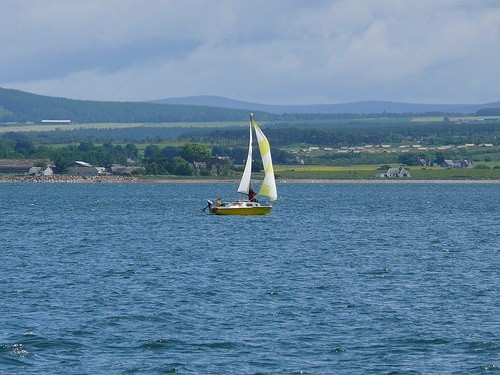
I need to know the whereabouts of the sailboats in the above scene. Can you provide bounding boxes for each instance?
[206,113,277,216]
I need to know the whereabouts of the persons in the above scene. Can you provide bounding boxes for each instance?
[248,187,259,203]
